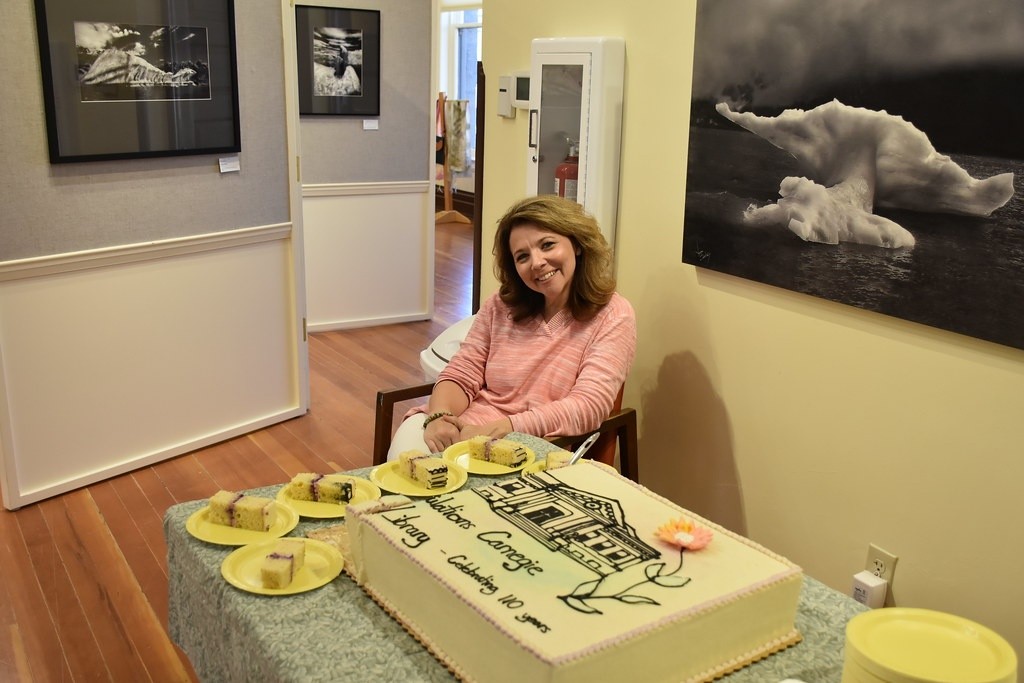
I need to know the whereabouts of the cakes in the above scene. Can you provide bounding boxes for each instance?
[545,450,575,469]
[468,434,527,468]
[208,489,278,533]
[258,539,305,590]
[289,472,358,505]
[397,449,448,489]
[346,459,805,683]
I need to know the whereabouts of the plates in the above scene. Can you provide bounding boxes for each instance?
[276,475,381,519]
[185,503,299,546]
[442,440,535,475]
[371,460,468,496]
[842,607,1019,683]
[521,459,617,477]
[221,537,344,595]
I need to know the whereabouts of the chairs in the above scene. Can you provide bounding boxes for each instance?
[372,376,639,484]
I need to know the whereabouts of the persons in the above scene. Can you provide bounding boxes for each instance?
[387,194,636,460]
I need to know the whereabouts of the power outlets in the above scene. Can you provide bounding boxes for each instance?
[867,543,898,594]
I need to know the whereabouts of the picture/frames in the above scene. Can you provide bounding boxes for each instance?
[34,0,242,165]
[294,3,381,117]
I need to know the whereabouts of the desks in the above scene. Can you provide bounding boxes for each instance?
[162,431,876,683]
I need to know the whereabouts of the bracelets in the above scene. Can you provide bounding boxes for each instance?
[423,411,453,428]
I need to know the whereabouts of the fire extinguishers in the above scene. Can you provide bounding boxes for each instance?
[554,136,579,201]
